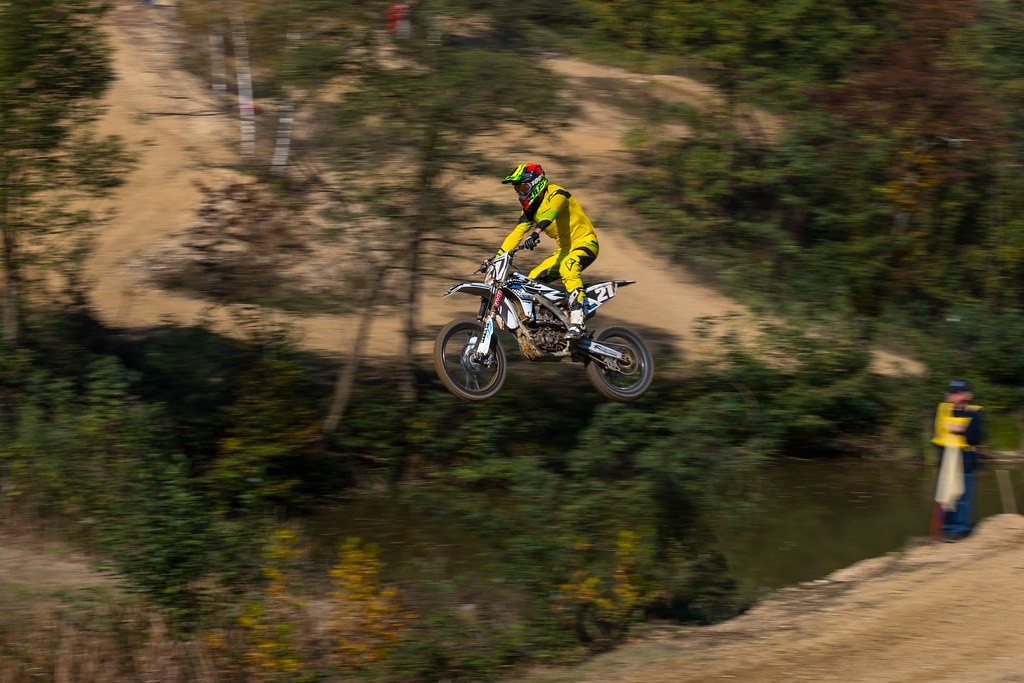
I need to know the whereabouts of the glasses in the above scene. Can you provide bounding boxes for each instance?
[514,171,545,196]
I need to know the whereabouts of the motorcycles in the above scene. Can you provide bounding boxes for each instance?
[433,236,656,404]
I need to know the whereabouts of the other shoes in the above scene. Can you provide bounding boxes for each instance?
[943,531,970,542]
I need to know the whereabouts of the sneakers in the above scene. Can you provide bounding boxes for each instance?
[508,320,539,333]
[564,324,589,340]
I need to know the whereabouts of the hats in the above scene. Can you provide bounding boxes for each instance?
[947,378,973,393]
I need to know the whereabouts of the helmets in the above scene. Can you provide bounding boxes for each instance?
[502,163,548,210]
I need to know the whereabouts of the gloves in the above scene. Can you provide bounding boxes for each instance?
[524,232,540,251]
[480,258,492,273]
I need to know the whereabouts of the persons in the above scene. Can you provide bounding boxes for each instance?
[930,380,984,543]
[479,163,599,340]
